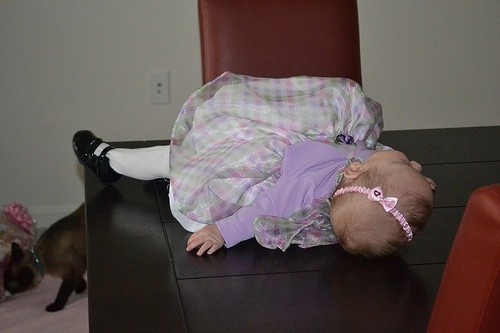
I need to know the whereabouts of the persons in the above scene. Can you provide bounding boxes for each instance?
[72,72,438,257]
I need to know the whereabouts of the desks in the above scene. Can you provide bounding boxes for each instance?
[84,126,500,333]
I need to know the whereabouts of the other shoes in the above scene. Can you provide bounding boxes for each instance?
[72,129,123,184]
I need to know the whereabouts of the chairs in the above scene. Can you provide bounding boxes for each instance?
[198,0,362,88]
[426,182,500,333]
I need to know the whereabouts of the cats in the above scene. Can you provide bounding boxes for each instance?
[3,203,87,312]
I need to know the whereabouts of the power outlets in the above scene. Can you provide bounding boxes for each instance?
[147,71,171,104]
